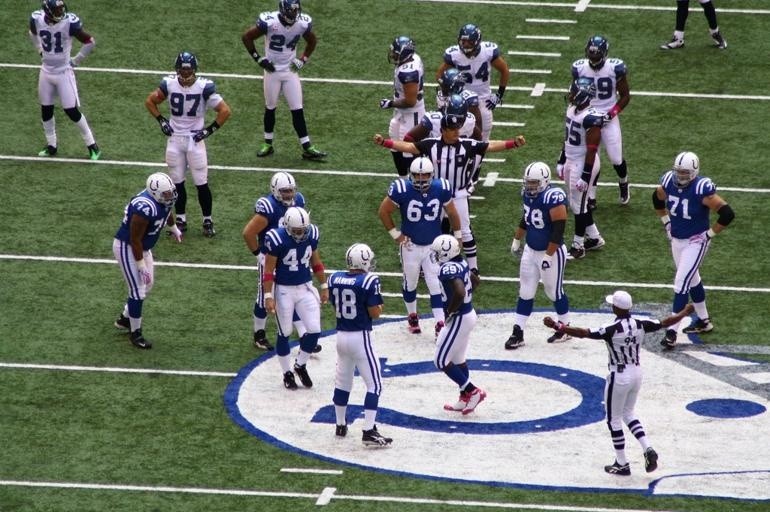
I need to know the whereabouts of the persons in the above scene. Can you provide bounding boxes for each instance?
[28,0,101,159]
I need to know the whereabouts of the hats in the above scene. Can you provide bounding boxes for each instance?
[605,289,633,311]
[439,114,465,130]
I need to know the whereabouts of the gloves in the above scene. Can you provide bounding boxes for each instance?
[509,243,525,262]
[539,256,553,272]
[379,98,391,110]
[688,230,708,247]
[665,221,675,243]
[190,128,213,143]
[555,163,565,182]
[575,179,590,195]
[169,224,183,244]
[484,93,502,112]
[292,58,306,73]
[258,55,278,74]
[138,266,154,286]
[159,114,175,139]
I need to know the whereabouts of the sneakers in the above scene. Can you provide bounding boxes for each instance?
[644,448,658,472]
[713,29,728,50]
[660,36,685,50]
[35,145,61,158]
[658,317,712,348]
[86,142,102,163]
[407,264,483,343]
[334,418,348,437]
[604,463,633,478]
[300,145,328,161]
[255,140,278,158]
[128,325,152,350]
[360,424,393,447]
[548,327,572,344]
[444,388,488,415]
[503,325,526,350]
[166,217,216,239]
[253,327,325,392]
[564,182,632,261]
[115,315,133,329]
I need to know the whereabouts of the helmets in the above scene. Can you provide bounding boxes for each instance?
[586,35,609,57]
[572,78,595,100]
[672,149,700,183]
[269,171,296,202]
[346,243,377,275]
[524,162,551,192]
[280,206,310,234]
[409,155,435,175]
[433,234,460,262]
[279,0,303,21]
[444,68,465,89]
[458,23,482,54]
[389,36,415,64]
[42,0,67,22]
[174,51,198,72]
[146,172,176,207]
